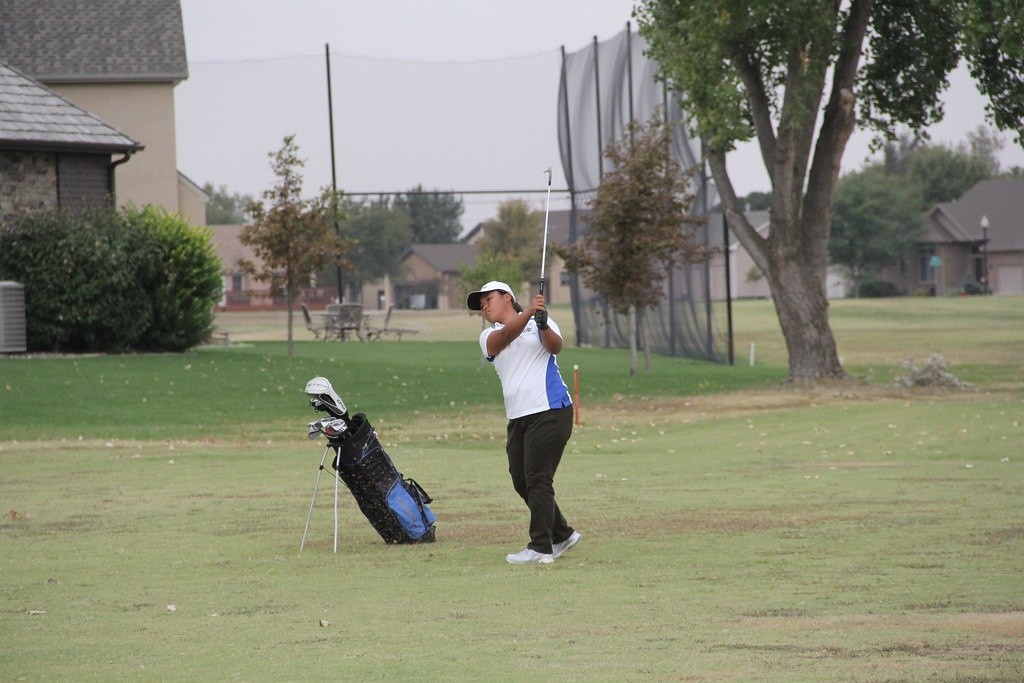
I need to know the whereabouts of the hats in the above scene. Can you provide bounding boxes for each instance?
[466,280,516,311]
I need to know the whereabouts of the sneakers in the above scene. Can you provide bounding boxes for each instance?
[551,532,580,558]
[506,546,553,563]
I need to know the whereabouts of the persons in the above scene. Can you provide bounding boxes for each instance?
[467,281,581,564]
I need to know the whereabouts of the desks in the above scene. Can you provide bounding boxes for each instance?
[315,312,374,341]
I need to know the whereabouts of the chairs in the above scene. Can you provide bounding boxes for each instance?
[300,302,419,343]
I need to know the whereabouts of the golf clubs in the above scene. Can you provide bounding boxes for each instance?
[536,166,554,327]
[306,416,352,440]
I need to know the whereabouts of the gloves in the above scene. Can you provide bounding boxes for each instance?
[534,307,549,330]
